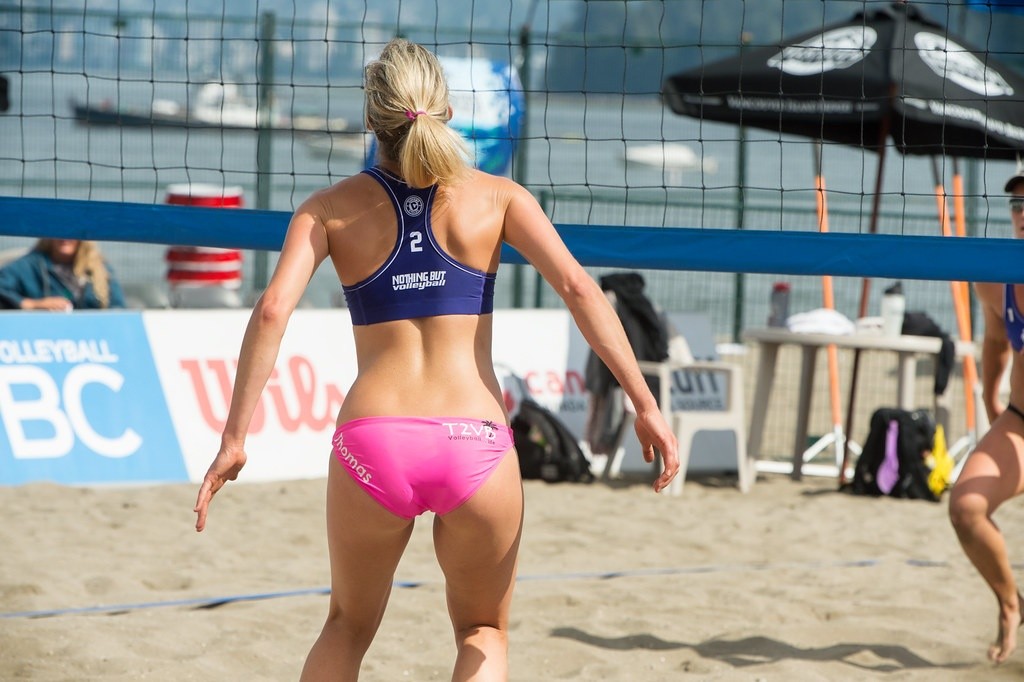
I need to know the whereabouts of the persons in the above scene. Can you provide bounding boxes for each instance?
[194,39,681,682]
[0,238,125,310]
[947,170,1024,662]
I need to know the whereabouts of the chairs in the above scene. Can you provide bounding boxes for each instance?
[600,291,747,498]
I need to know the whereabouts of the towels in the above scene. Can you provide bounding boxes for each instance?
[786,305,852,334]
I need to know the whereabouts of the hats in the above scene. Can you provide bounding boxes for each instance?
[1004,169,1024,192]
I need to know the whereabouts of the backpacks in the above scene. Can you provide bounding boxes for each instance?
[590,272,671,385]
[849,406,944,501]
[510,400,596,483]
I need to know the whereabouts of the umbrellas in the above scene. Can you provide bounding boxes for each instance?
[658,0,1024,487]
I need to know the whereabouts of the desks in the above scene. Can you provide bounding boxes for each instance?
[742,324,945,495]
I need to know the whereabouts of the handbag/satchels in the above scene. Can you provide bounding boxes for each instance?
[902,310,955,396]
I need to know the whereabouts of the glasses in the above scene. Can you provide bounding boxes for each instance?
[1009,198,1024,212]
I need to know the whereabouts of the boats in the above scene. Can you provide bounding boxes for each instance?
[616,140,717,176]
[67,89,348,136]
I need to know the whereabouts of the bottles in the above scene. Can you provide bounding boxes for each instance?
[767,283,791,330]
[880,279,906,335]
